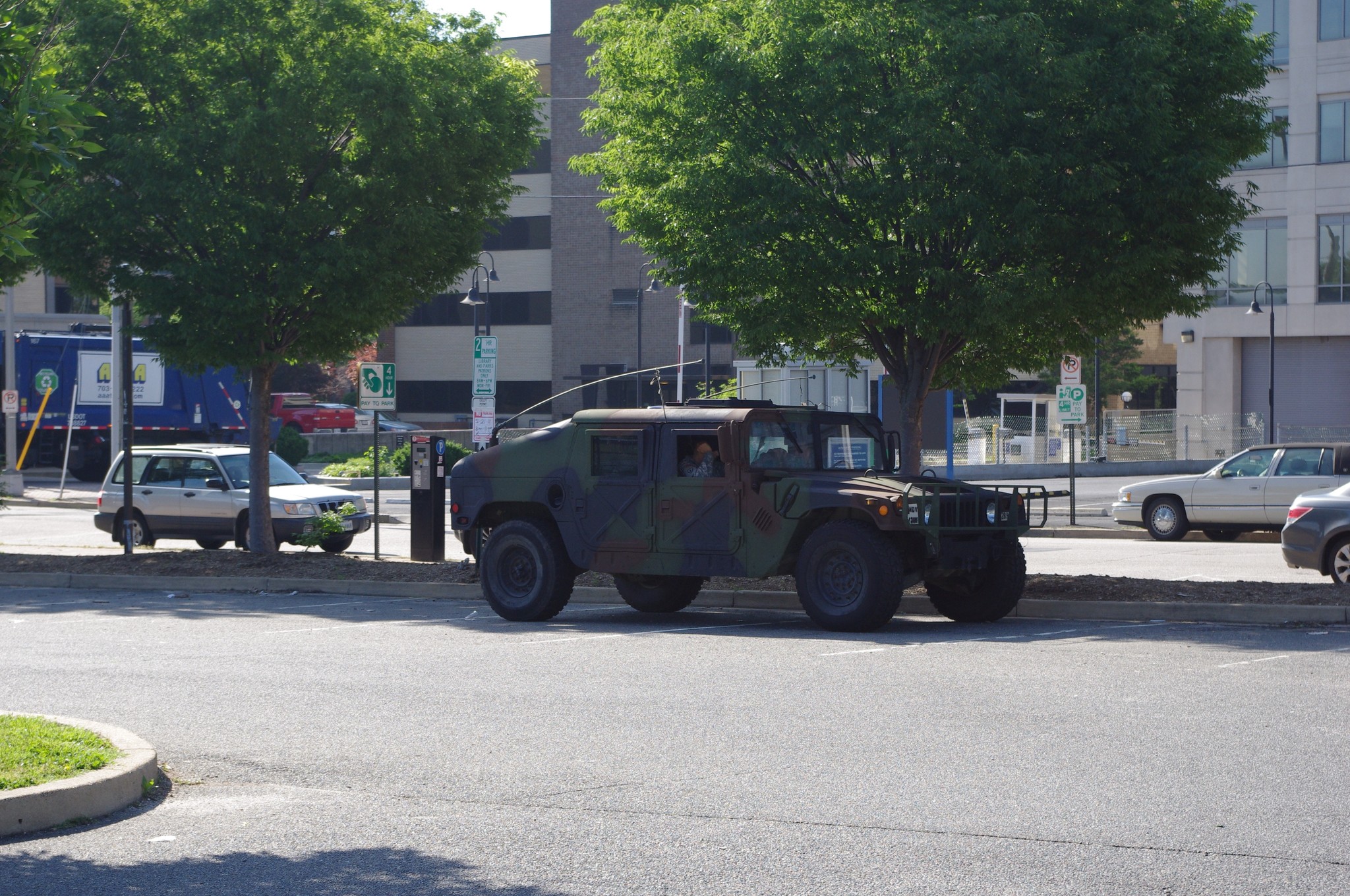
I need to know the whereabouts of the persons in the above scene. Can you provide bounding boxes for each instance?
[789,437,816,471]
[678,435,721,478]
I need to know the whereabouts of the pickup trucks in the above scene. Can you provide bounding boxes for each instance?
[267,392,354,433]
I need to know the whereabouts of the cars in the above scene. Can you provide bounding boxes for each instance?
[315,402,423,433]
[1111,441,1350,541]
[1281,481,1350,584]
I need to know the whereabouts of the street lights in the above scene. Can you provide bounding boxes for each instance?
[1244,281,1275,444]
[460,251,500,336]
[635,256,674,411]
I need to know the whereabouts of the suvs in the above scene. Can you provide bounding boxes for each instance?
[93,443,372,556]
[449,395,1050,630]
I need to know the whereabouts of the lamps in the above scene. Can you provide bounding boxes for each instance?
[1182,330,1196,345]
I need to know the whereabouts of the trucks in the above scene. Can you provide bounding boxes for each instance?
[14,322,280,483]
